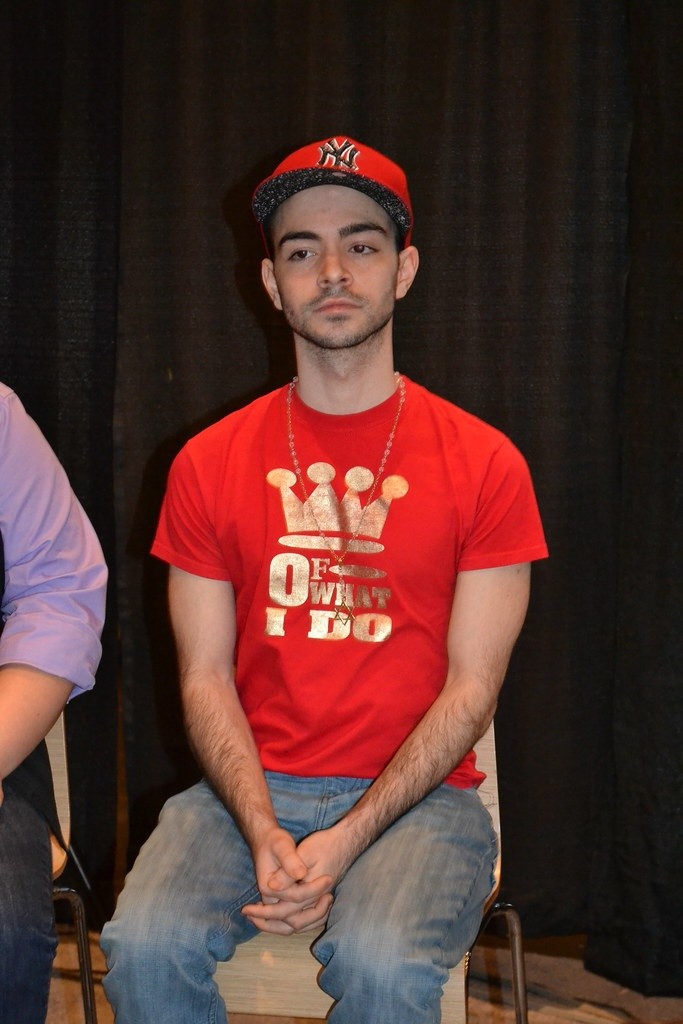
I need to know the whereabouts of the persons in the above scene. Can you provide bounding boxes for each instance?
[1,380,111,1024]
[101,134,549,1023]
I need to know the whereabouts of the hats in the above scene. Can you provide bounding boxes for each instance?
[251,137,414,260]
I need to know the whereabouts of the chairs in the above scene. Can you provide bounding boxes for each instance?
[211,715,529,1024]
[32,712,98,1024]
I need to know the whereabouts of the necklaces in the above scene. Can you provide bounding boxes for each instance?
[286,370,408,630]
